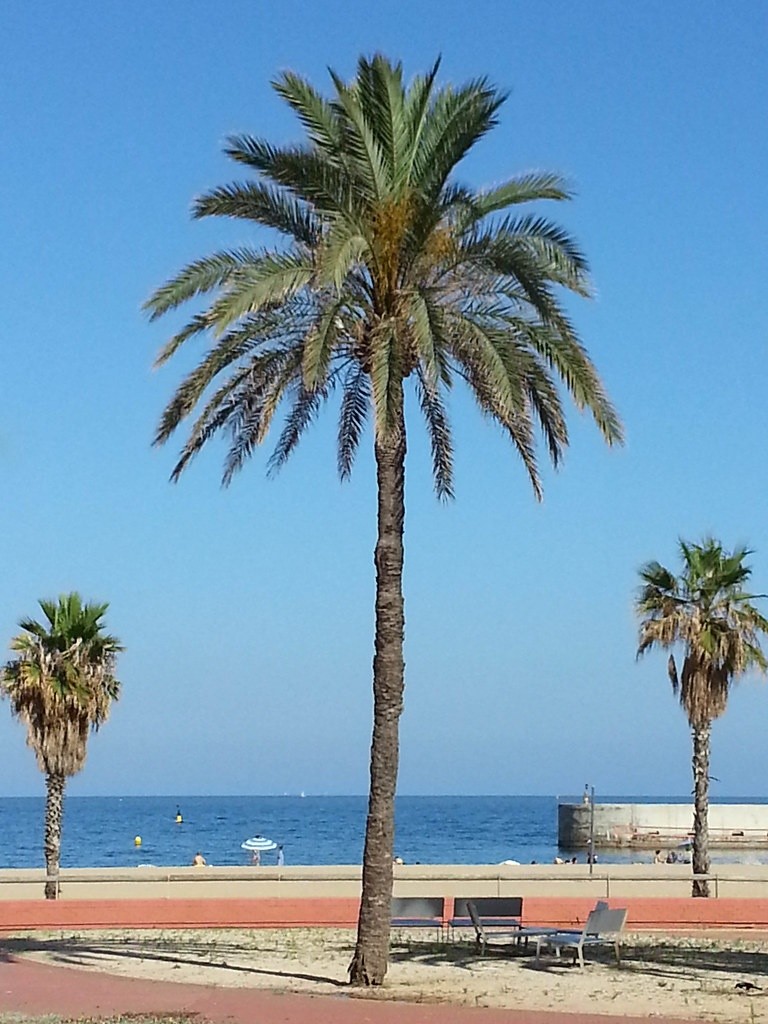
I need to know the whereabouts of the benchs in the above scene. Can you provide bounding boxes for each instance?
[447,897,523,947]
[390,897,446,949]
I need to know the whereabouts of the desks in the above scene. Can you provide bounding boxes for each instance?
[514,926,557,954]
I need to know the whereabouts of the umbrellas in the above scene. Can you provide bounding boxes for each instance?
[241,833,277,852]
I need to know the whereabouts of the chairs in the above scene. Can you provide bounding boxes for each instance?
[536,908,628,971]
[466,901,518,956]
[555,900,610,959]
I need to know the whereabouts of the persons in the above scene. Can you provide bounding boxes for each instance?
[193,852,207,867]
[252,846,286,866]
[393,850,693,865]
